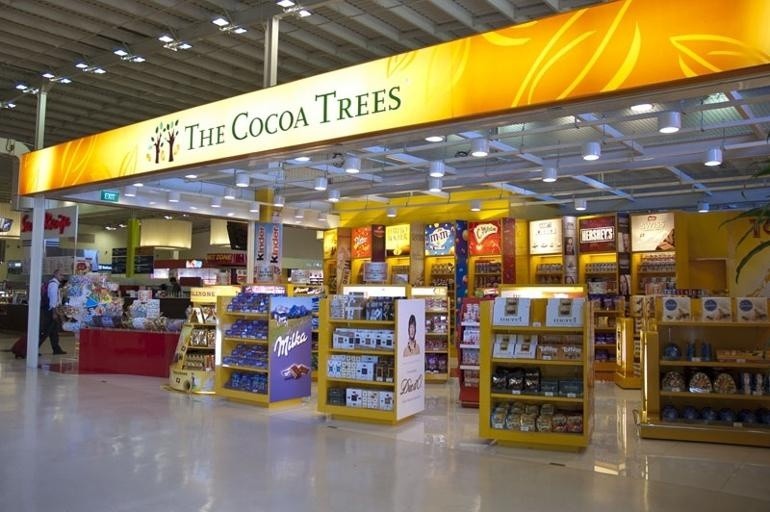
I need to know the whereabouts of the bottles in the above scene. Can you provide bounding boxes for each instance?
[538,262,616,272]
[688,341,712,361]
[0,292,9,304]
[639,254,675,271]
[432,263,454,274]
[184,354,204,370]
[475,259,490,273]
[393,265,409,273]
[664,286,678,295]
[343,292,367,320]
[168,290,183,298]
[540,275,560,284]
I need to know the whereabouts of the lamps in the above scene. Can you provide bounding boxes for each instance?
[124,109,725,218]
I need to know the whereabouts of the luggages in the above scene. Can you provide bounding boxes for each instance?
[11,330,43,359]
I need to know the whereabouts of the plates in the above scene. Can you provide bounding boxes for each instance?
[660,404,770,428]
[663,343,681,361]
[661,371,738,395]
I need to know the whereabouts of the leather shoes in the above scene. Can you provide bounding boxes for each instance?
[39,353,42,357]
[53,348,68,356]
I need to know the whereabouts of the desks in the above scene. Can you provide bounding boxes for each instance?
[0,293,191,378]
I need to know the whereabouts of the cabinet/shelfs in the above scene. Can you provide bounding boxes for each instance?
[459,219,594,456]
[169,208,465,428]
[578,212,770,448]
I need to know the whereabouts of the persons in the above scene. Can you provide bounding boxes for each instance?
[403,315,420,357]
[39,268,68,356]
[169,277,181,297]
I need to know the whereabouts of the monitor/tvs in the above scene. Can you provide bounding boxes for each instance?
[226,220,248,250]
